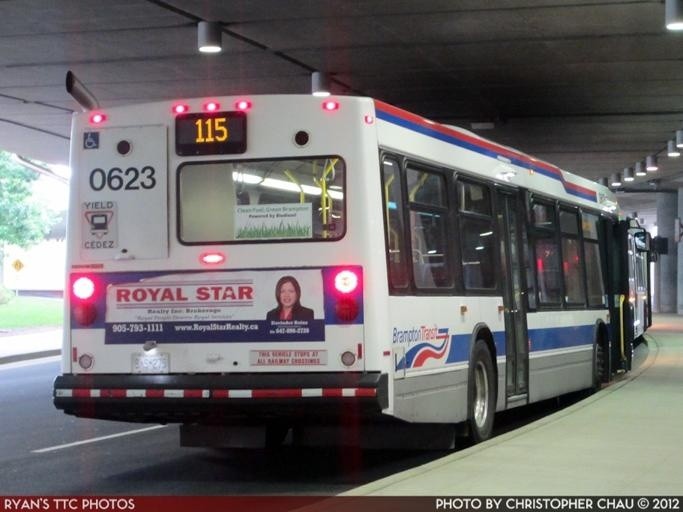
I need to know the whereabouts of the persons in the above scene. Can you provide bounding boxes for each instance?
[267,276,313,322]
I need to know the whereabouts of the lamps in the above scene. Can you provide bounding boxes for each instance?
[596,128,682,188]
[196,20,224,55]
[664,0,682,31]
[310,70,331,99]
